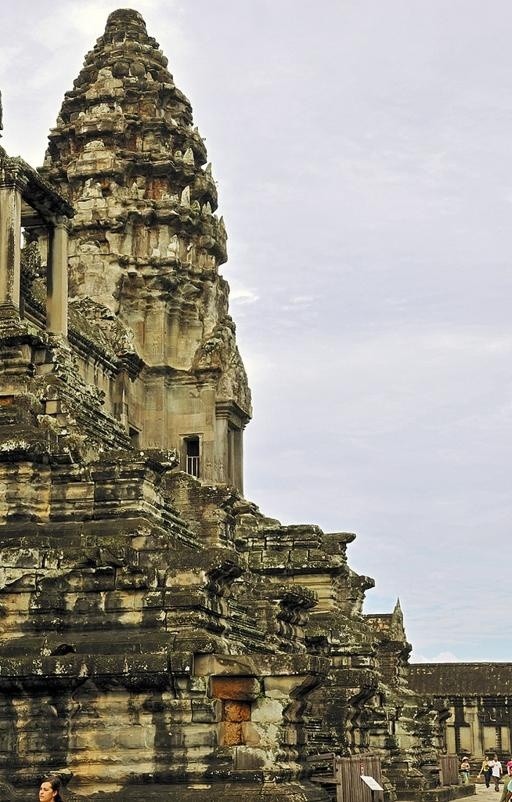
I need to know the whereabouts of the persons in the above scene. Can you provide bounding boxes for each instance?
[500,761,512,802]
[461,756,470,784]
[479,755,503,792]
[39,777,64,802]
